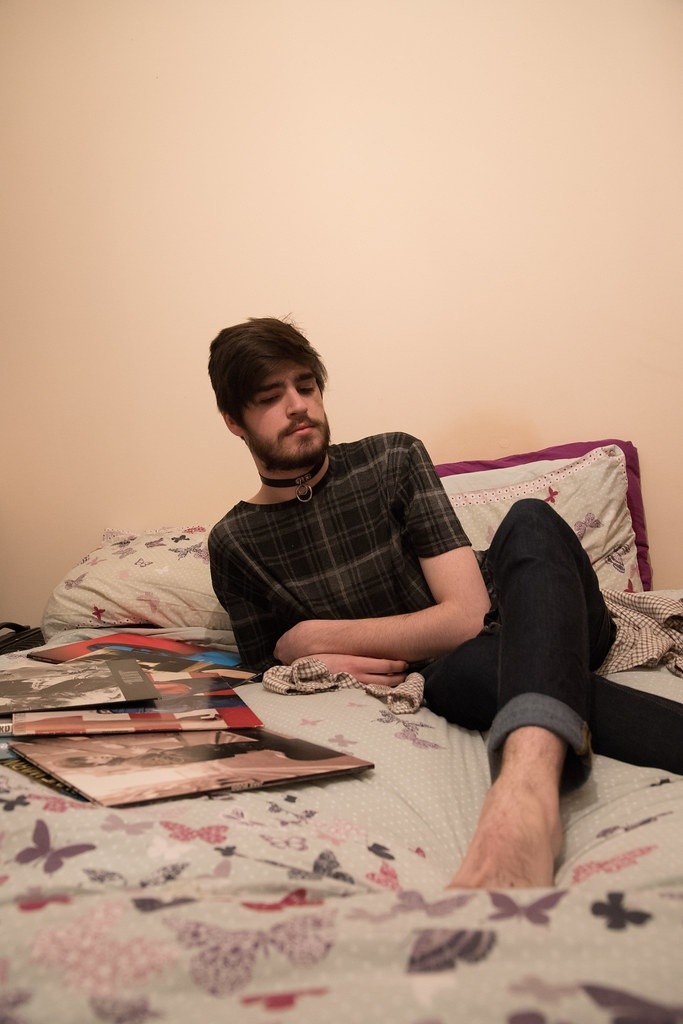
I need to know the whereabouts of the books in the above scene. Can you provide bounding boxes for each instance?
[0,632,376,810]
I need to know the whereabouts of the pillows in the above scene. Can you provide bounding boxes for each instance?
[40,526,235,642]
[435,440,654,594]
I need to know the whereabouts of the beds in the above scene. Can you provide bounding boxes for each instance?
[0,624,683,1024]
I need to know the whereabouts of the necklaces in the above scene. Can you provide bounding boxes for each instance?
[259,455,326,503]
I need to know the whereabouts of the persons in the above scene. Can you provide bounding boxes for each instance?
[207,316,683,889]
[97,691,248,714]
[58,735,281,768]
